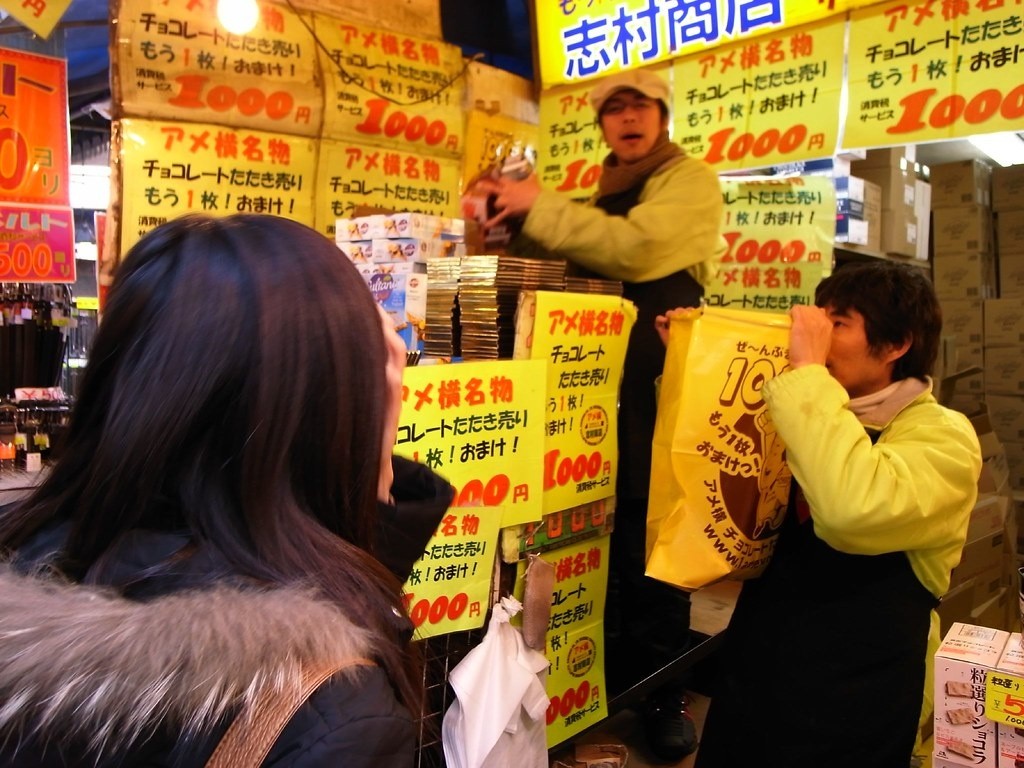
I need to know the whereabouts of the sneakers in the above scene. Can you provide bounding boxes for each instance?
[648,686,698,759]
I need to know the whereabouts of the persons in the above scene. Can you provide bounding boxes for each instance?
[694,263,982,768]
[0,214,455,768]
[484,70,721,758]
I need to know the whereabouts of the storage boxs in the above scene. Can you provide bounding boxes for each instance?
[333,204,467,366]
[779,143,1024,768]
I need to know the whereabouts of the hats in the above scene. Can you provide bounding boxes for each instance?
[589,67,671,111]
